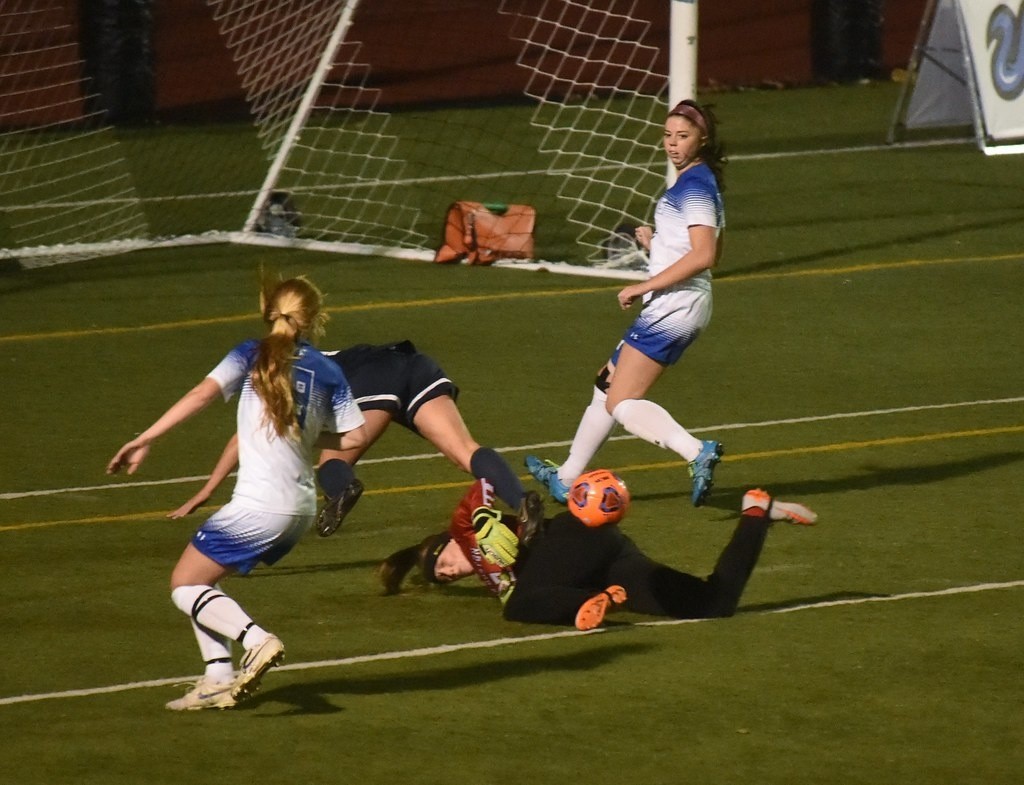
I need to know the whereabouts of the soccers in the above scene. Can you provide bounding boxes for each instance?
[569,469,630,529]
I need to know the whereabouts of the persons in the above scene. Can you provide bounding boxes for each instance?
[521,96,733,508]
[300,337,523,533]
[377,470,818,632]
[103,274,368,711]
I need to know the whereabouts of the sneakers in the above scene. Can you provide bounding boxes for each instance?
[233,635,286,701]
[739,487,818,525]
[522,454,569,504]
[687,439,725,507]
[574,584,628,629]
[163,679,239,710]
[313,478,364,535]
